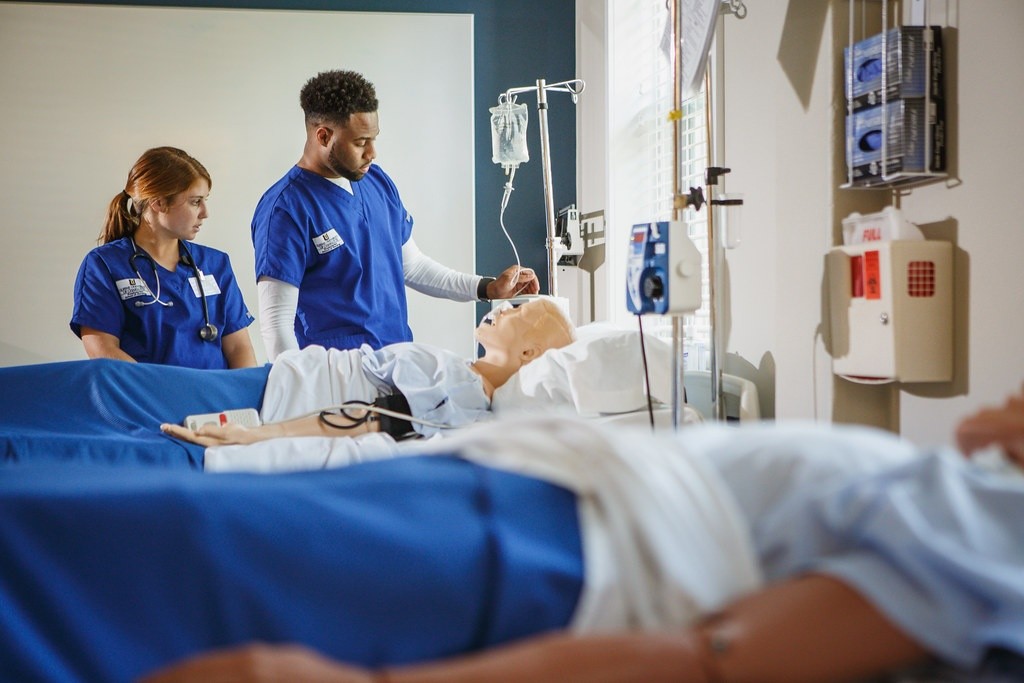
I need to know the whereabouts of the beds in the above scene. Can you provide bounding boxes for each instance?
[0,363,769,473]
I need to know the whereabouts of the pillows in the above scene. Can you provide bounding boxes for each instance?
[488,319,689,419]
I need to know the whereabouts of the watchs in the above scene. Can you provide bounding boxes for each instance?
[477,276,496,304]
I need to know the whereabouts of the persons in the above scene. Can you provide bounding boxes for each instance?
[0,296,578,449]
[1,381,1024,683]
[251,70,542,362]
[69,146,258,370]
